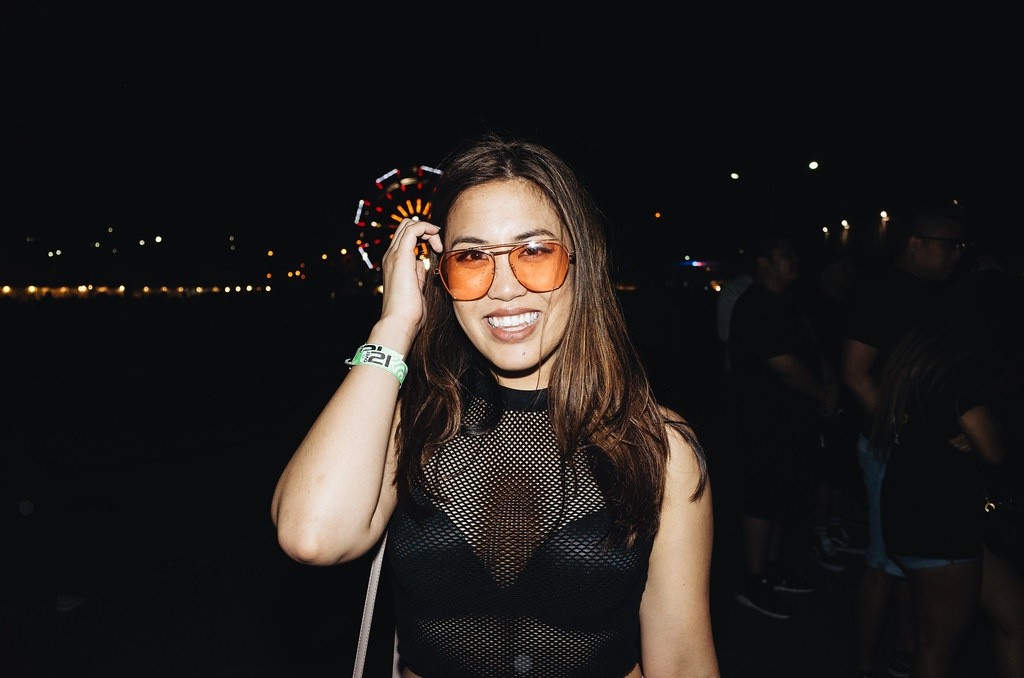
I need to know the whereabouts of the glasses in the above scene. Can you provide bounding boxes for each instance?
[433,239,577,301]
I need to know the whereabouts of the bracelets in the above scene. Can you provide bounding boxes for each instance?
[346,345,407,386]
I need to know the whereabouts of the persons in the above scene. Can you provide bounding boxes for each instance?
[715,208,1024,678]
[272,142,721,678]
